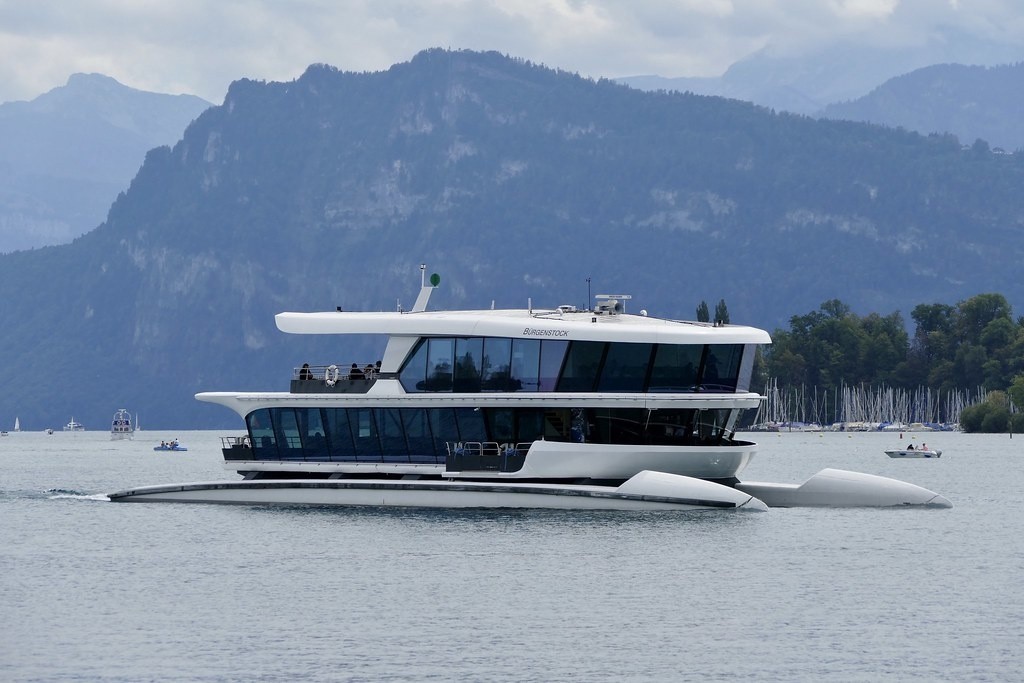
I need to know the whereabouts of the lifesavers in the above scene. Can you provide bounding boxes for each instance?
[325,364,339,386]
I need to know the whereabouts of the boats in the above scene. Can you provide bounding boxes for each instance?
[883,448,943,458]
[153,444,187,451]
[107,261,954,514]
[110,408,135,441]
[1,430,9,436]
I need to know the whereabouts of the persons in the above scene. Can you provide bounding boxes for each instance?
[375,360,382,373]
[300,364,313,380]
[349,363,366,380]
[161,438,179,449]
[922,443,928,451]
[907,443,914,451]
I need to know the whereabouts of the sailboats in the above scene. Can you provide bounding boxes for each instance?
[738,377,988,432]
[13,417,21,432]
[134,413,140,431]
